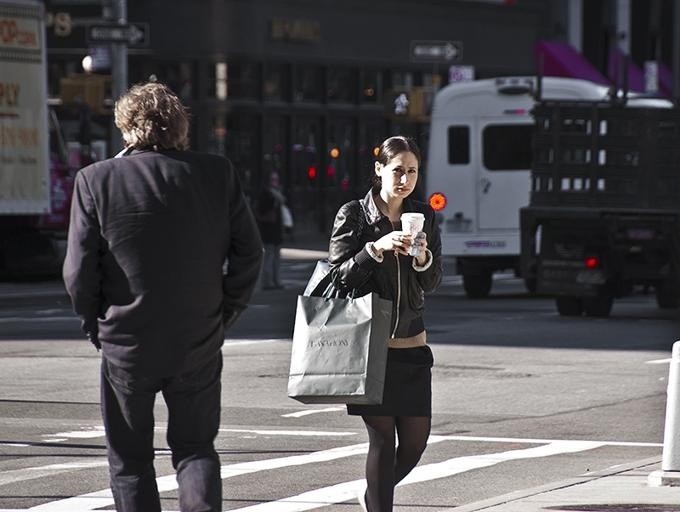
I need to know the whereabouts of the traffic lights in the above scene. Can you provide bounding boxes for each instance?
[387,86,424,123]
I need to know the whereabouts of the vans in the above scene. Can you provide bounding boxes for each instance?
[427,75,673,298]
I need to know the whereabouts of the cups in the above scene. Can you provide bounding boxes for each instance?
[400,212,426,254]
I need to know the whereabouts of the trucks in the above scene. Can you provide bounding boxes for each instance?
[519,56,680,317]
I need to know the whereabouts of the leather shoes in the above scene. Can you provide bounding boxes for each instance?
[358,487,368,512]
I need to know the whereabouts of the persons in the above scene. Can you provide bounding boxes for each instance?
[253,172,293,290]
[329,135,444,512]
[62,81,265,512]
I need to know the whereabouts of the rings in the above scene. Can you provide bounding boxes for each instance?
[398,236,401,241]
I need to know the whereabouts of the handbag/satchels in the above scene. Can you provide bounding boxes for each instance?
[287,261,393,407]
